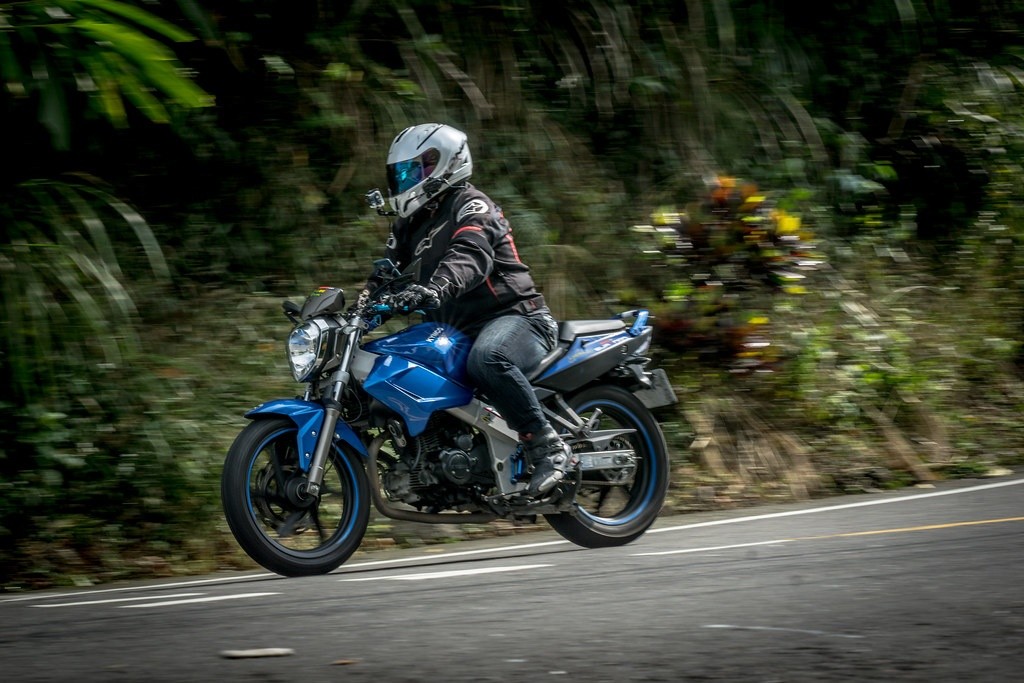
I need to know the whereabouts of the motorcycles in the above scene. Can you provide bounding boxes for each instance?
[222,260,682,577]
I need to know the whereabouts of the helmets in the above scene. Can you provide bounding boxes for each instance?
[385,122,473,219]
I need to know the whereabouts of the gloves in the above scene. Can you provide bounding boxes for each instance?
[386,284,438,314]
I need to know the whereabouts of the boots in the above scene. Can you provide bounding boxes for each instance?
[520,421,573,496]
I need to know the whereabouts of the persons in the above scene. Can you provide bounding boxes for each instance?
[361,123,571,495]
